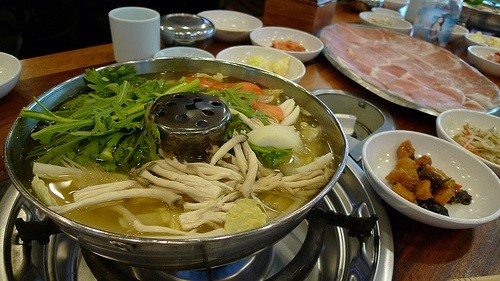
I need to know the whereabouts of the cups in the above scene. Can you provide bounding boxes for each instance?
[108,7,161,63]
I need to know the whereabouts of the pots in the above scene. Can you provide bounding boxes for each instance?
[3,57,349,271]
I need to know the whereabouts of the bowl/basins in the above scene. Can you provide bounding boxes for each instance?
[372,7,403,20]
[250,26,330,64]
[153,47,214,63]
[359,11,413,36]
[216,46,306,85]
[384,0,407,10]
[464,34,500,49]
[467,45,500,79]
[362,130,500,228]
[0,51,21,100]
[448,24,469,43]
[197,9,263,42]
[437,109,500,178]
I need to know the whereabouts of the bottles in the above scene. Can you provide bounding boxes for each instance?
[410,0,464,48]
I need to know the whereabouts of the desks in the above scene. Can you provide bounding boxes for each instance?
[0,0,500,281]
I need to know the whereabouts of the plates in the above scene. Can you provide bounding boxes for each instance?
[319,21,500,115]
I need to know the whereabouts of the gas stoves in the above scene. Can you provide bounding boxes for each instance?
[0,154,394,281]
